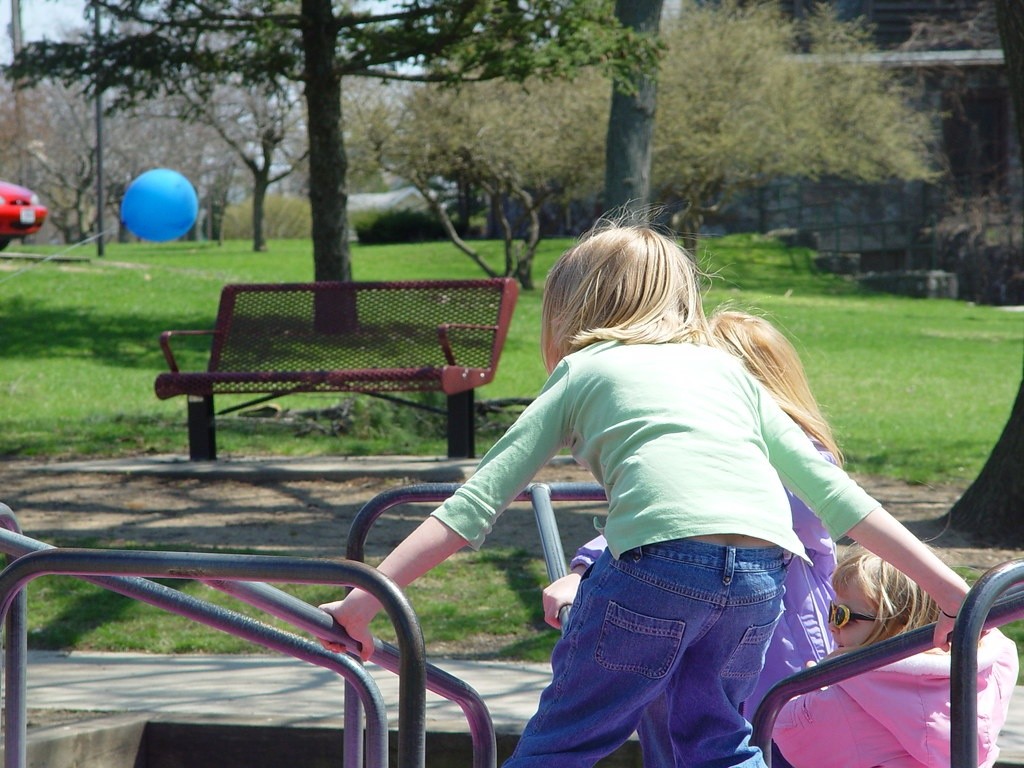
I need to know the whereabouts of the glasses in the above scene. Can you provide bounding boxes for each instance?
[827,601,876,628]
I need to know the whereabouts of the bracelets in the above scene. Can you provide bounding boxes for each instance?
[941,608,957,621]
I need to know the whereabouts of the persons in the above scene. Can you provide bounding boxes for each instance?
[315,197,969,768]
[541,298,846,768]
[771,517,1020,768]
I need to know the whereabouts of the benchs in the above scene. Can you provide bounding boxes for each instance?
[153,277,519,460]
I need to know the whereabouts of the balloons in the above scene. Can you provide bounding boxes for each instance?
[116,165,200,245]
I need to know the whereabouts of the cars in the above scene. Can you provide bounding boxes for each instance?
[0,180,49,253]
